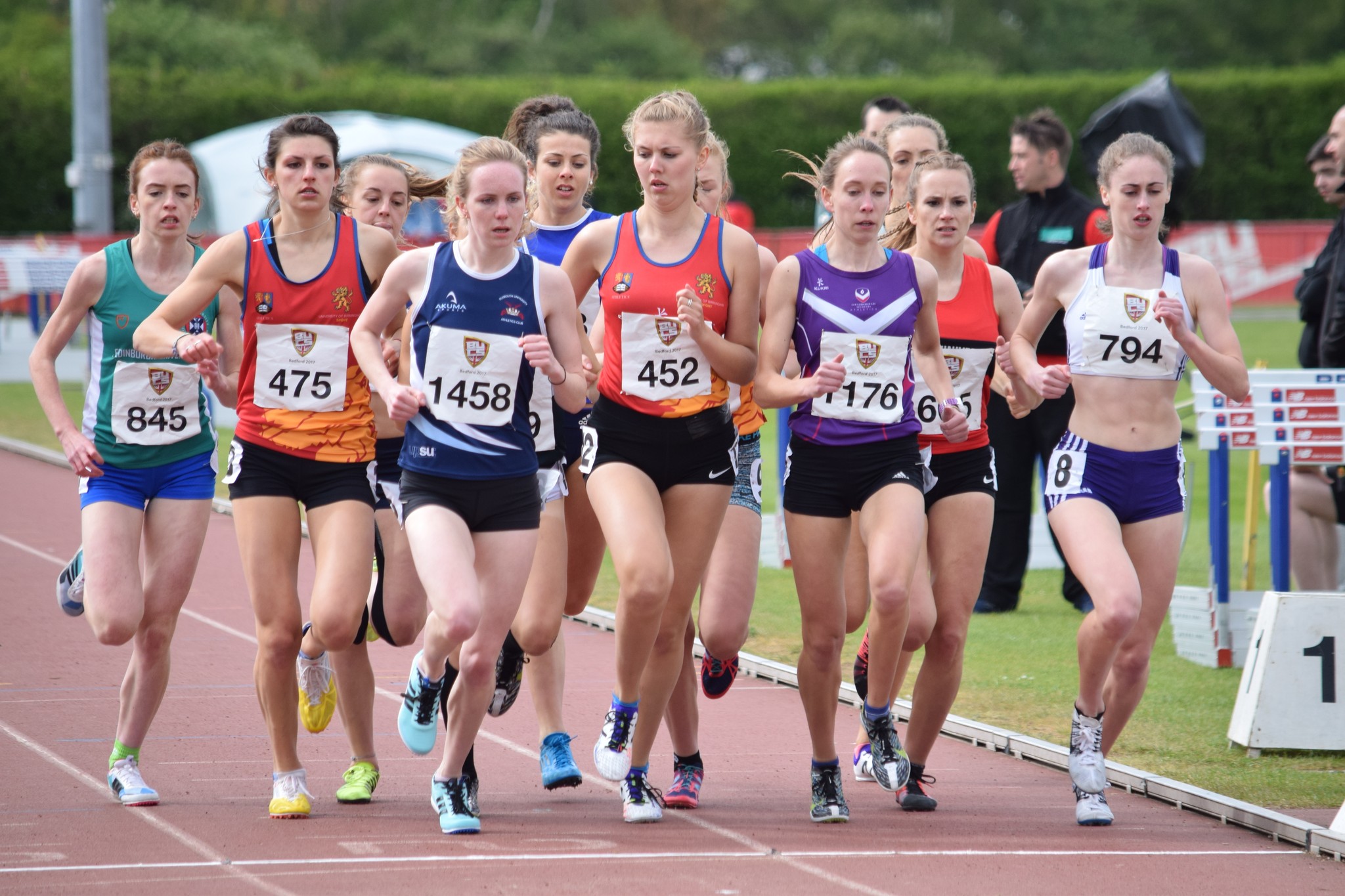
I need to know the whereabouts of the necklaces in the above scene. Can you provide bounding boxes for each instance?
[252,207,331,242]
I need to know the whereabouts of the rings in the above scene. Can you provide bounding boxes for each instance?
[184,344,195,350]
[387,359,391,368]
[686,298,692,307]
[194,340,204,346]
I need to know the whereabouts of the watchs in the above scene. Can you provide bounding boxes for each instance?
[938,397,969,417]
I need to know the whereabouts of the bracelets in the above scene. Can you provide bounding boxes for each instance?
[170,331,199,361]
[547,366,566,385]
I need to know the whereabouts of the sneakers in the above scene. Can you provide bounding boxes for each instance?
[488,647,530,718]
[619,769,668,823]
[297,620,338,733]
[335,762,380,804]
[895,770,938,811]
[859,693,911,791]
[56,543,84,617]
[810,765,849,822]
[397,648,446,755]
[852,744,878,781]
[1072,782,1115,827]
[268,768,315,819]
[662,757,704,809]
[429,767,482,835]
[592,700,639,780]
[700,647,739,699]
[853,626,870,699]
[1067,698,1106,794]
[107,754,159,807]
[538,731,582,792]
[365,555,381,643]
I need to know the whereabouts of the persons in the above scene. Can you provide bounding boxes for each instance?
[331,135,591,838]
[751,95,1115,827]
[694,138,728,216]
[1008,130,1250,827]
[1260,96,1345,608]
[26,140,246,809]
[496,89,783,830]
[132,114,400,820]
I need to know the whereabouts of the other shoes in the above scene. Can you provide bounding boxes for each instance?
[972,597,1019,613]
[1073,593,1095,616]
